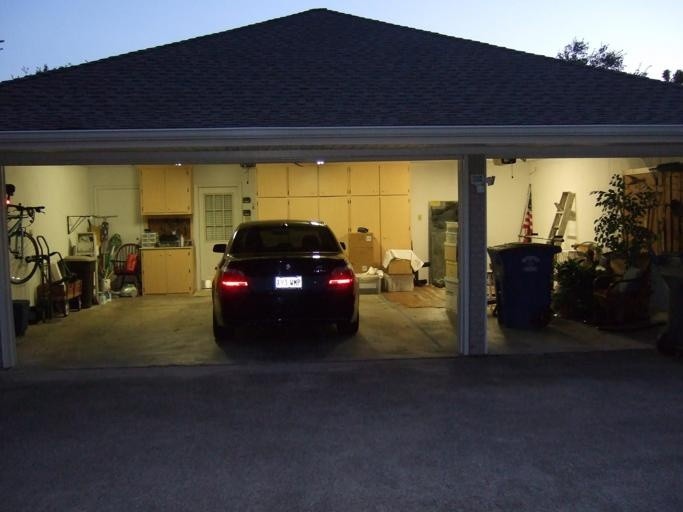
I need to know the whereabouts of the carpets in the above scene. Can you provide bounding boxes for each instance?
[383,285,446,308]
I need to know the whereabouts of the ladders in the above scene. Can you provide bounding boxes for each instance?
[546,192,576,262]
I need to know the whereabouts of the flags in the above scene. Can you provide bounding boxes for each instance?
[521,187,532,244]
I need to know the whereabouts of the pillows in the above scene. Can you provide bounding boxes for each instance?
[620,265,640,293]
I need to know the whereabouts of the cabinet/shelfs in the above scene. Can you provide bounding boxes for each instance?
[257,161,411,270]
[139,246,198,295]
[137,164,194,215]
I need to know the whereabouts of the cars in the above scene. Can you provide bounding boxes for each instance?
[208,217,361,343]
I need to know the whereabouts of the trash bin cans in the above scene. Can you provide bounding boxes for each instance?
[486,242,562,330]
[62,256,97,309]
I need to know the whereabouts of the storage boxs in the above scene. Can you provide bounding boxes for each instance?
[357,249,414,293]
[444,221,458,278]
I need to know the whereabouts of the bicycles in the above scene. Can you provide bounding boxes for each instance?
[6,201,45,285]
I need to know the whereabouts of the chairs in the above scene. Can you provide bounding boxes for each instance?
[591,253,653,323]
